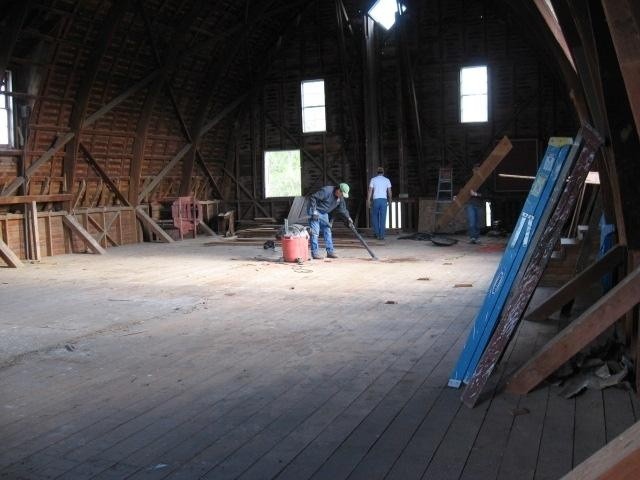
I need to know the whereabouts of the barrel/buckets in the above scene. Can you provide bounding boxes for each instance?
[281,234,309,262]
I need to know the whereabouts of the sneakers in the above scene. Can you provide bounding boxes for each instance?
[313,253,325,259]
[327,253,339,258]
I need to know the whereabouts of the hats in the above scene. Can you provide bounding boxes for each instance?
[336,182,350,199]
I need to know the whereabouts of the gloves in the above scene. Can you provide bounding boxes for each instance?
[312,212,320,222]
[344,218,353,228]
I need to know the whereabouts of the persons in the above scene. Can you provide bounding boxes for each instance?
[306,181,354,261]
[453,162,497,245]
[364,166,395,242]
